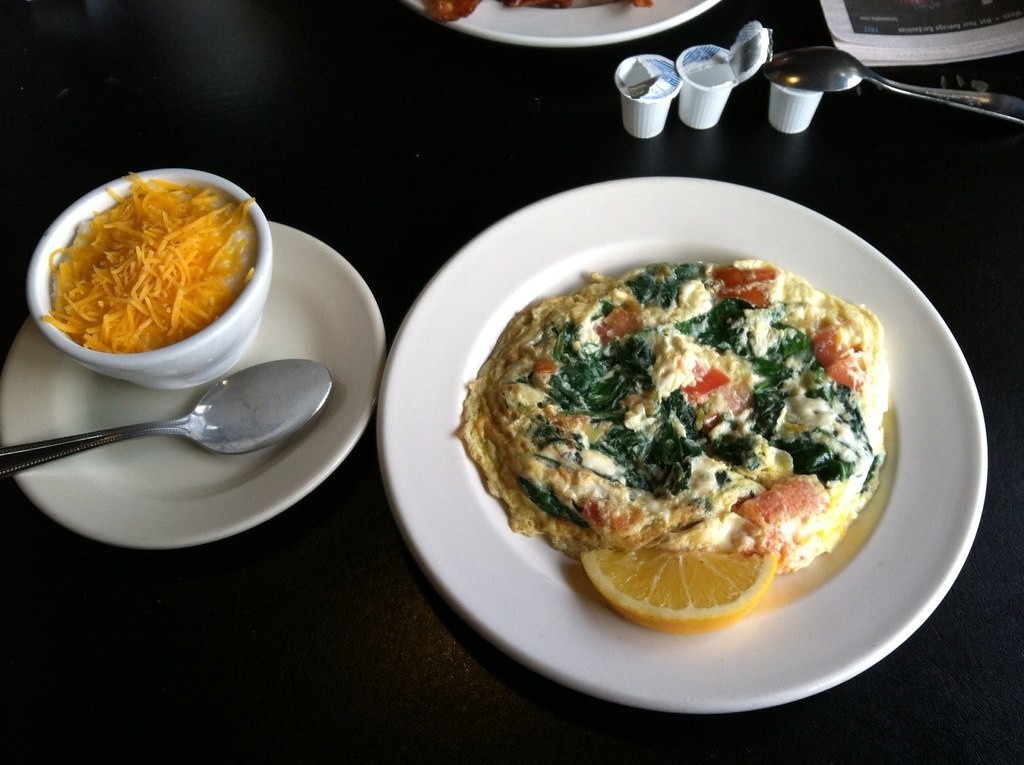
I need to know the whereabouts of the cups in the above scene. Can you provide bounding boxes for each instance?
[767,80,824,134]
[23,167,277,390]
[675,46,739,129]
[613,55,682,139]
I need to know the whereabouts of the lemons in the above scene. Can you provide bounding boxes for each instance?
[578,547,777,635]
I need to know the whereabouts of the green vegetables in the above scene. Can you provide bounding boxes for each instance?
[516,262,886,530]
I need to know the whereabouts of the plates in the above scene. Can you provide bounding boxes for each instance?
[0,217,387,551]
[376,175,989,715]
[400,0,721,47]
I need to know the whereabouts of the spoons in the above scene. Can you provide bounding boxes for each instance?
[0,359,335,488]
[762,46,1024,127]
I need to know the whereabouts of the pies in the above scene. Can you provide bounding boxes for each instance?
[458,257,889,577]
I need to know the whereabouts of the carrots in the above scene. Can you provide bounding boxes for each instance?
[39,172,257,354]
